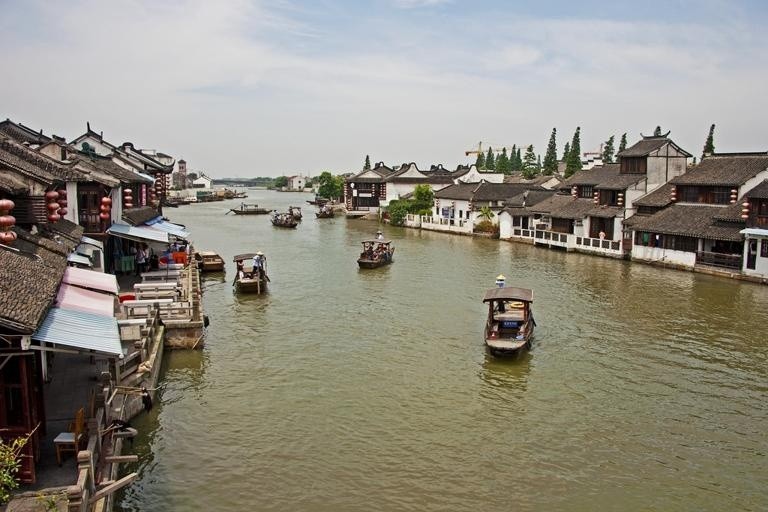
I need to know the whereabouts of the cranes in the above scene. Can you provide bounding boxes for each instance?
[466,141,536,158]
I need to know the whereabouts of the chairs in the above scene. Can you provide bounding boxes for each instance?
[53,407,85,467]
[67,388,96,444]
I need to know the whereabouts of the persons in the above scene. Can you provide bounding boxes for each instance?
[496,274,505,289]
[273,206,300,225]
[598,230,606,240]
[365,230,391,261]
[241,202,243,211]
[129,240,189,277]
[238,252,264,279]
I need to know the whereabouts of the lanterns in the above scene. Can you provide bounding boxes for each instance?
[741,201,750,222]
[123,186,133,210]
[730,189,738,206]
[0,195,18,246]
[468,200,472,211]
[343,184,348,197]
[593,191,599,208]
[435,198,439,208]
[617,192,624,208]
[670,188,677,202]
[46,185,68,224]
[451,201,455,211]
[149,171,170,207]
[99,197,112,223]
[372,184,386,198]
[573,186,577,200]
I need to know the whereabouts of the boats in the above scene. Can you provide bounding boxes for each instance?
[202,251,226,272]
[163,189,248,208]
[482,287,536,354]
[231,254,271,294]
[305,196,335,218]
[271,212,298,228]
[287,206,303,218]
[357,238,395,269]
[230,204,273,215]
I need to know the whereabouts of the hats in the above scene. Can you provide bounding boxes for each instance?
[497,273,505,280]
[377,230,381,234]
[256,250,263,255]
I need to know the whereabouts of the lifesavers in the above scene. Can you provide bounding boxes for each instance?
[511,301,524,309]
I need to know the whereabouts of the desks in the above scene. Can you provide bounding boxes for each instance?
[123,264,185,320]
